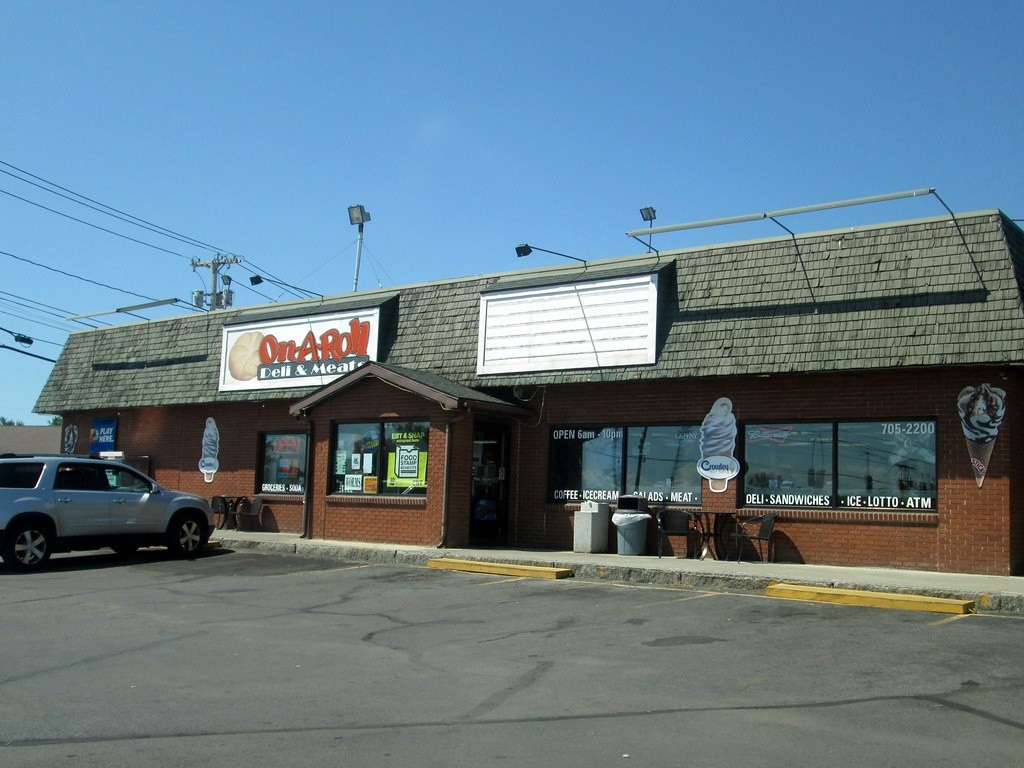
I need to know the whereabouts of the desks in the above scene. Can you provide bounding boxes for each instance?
[214,495,247,530]
[692,509,735,563]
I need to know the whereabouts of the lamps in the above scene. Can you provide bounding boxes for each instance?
[249,275,324,298]
[640,206,658,253]
[346,205,371,291]
[514,245,588,266]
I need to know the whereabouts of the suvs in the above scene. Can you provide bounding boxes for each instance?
[0,453,214,571]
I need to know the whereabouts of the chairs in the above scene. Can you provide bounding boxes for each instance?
[656,508,700,559]
[236,497,264,532]
[210,496,233,530]
[729,511,780,565]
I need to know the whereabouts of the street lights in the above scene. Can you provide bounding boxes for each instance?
[349,204,371,292]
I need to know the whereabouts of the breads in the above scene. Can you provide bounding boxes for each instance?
[228,331,264,382]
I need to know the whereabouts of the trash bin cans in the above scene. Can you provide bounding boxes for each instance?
[611,494,653,556]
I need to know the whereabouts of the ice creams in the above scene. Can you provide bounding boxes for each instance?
[696,397,740,493]
[956,382,1007,487]
[64,425,78,454]
[198,417,220,482]
